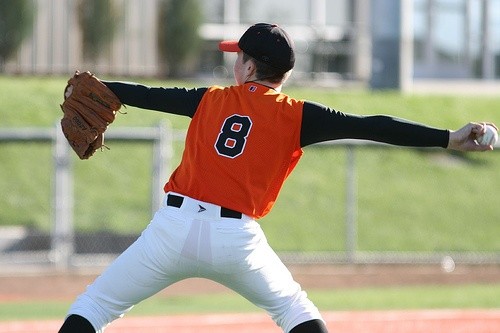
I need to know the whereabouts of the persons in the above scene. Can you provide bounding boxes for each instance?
[58,24,500,333]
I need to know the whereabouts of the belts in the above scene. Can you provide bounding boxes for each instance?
[167,194,243,220]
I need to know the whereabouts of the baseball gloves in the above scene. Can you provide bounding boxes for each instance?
[60,69,126,159]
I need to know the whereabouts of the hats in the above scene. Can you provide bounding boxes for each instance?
[219,22,296,71]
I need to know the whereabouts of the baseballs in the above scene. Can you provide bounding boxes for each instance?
[476,125,498,146]
